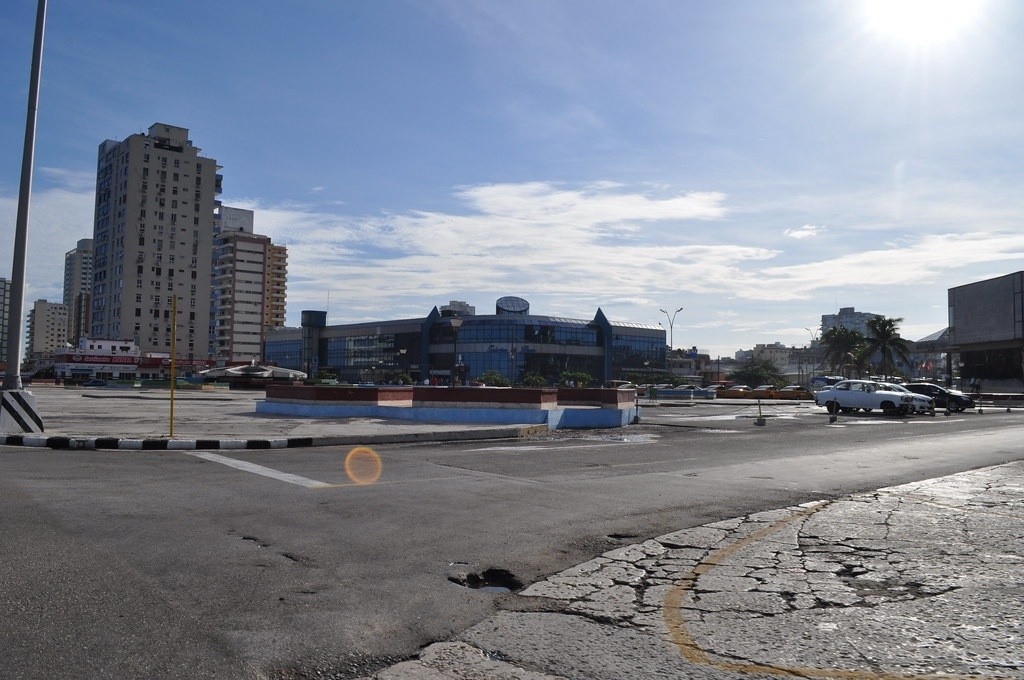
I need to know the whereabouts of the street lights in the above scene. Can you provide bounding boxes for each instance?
[798,352,807,385]
[717,355,720,384]
[868,364,872,380]
[194,354,203,371]
[958,360,965,391]
[643,360,650,392]
[400,347,407,385]
[659,307,683,375]
[804,327,821,379]
[450,312,464,387]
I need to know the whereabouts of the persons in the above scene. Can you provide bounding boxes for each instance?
[565,379,575,388]
[945,374,950,387]
[439,376,448,386]
[432,377,438,386]
[398,380,403,386]
[969,377,982,392]
[424,378,429,384]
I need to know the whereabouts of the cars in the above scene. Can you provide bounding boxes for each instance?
[728,385,753,398]
[636,384,655,395]
[653,384,674,389]
[359,381,376,387]
[470,381,486,387]
[894,377,903,384]
[321,374,343,384]
[820,383,935,414]
[753,385,780,399]
[602,380,632,388]
[897,382,975,413]
[673,385,699,391]
[617,384,638,389]
[779,386,814,400]
[83,379,107,386]
[870,376,881,382]
[701,385,729,398]
[815,380,911,416]
